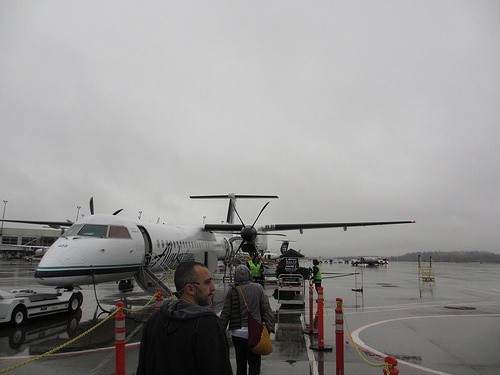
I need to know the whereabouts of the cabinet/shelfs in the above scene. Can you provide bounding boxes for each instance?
[275,274,305,341]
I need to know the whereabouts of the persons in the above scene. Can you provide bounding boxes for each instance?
[135,260,233,375]
[309,259,322,293]
[245,255,264,288]
[219,264,274,375]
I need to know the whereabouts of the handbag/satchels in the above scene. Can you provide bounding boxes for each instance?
[247,312,273,355]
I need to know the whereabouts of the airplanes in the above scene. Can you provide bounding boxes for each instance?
[0,194,416,300]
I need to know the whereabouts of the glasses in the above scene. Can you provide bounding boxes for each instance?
[173,281,200,297]
